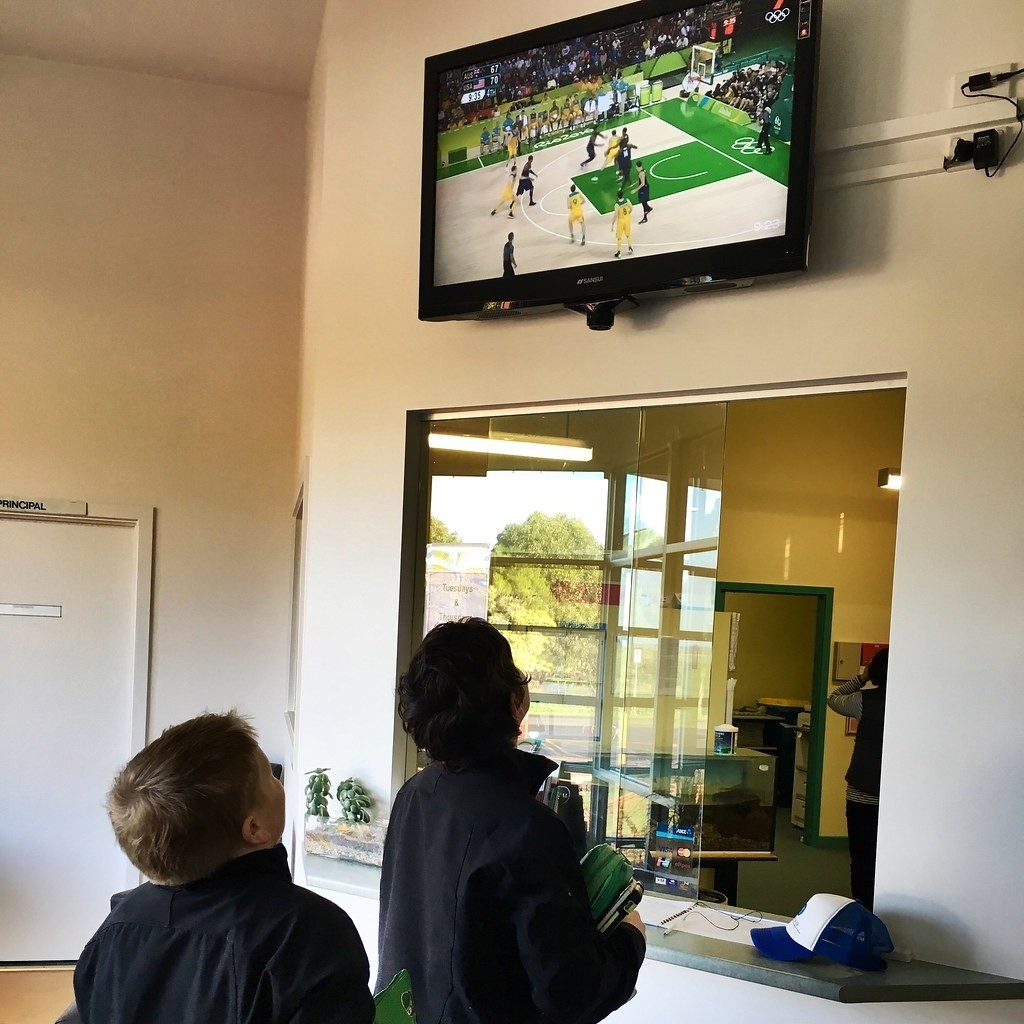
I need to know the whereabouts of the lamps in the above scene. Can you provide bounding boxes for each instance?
[428,413,594,463]
[877,467,901,490]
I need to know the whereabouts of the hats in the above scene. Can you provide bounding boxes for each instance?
[750,893,895,971]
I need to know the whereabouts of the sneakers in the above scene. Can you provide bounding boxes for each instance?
[646,208,653,213]
[581,239,585,244]
[508,214,514,218]
[628,248,633,253]
[491,210,496,215]
[615,253,621,257]
[639,218,647,224]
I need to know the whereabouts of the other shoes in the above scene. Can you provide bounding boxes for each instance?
[480,119,596,156]
[528,202,536,206]
[764,150,771,154]
[755,145,762,149]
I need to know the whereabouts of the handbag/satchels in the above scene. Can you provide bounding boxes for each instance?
[579,843,634,922]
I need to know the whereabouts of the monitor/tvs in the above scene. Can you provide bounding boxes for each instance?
[418,0,823,330]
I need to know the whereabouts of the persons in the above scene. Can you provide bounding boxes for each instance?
[827,650,888,912]
[436,0,790,278]
[372,615,648,1023]
[73,712,377,1024]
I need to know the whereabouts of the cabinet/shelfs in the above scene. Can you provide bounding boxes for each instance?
[733,715,786,751]
[790,712,810,830]
